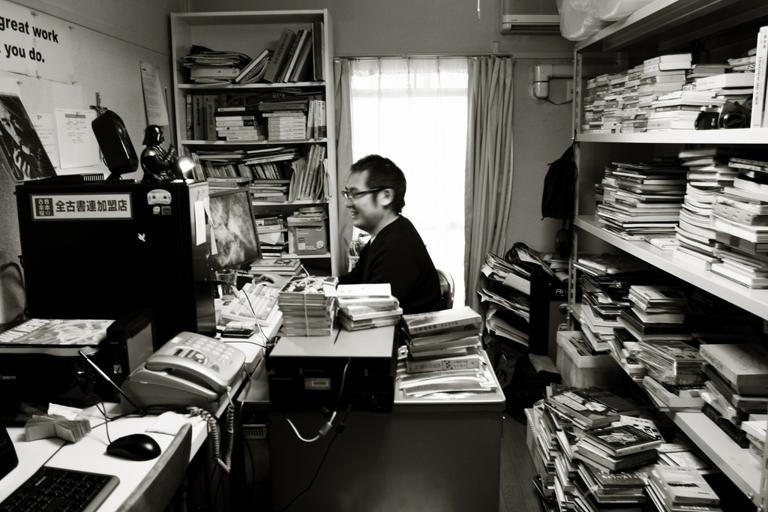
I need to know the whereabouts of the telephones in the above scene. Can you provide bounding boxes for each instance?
[77,331,247,417]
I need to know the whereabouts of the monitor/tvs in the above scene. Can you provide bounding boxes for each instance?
[205,188,264,296]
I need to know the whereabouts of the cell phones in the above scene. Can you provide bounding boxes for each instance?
[222,326,255,340]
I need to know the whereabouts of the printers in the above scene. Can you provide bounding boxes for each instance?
[0,315,154,405]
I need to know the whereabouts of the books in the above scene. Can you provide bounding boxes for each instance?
[594,149,768,290]
[276,266,490,400]
[524,333,720,512]
[476,242,568,346]
[178,24,329,272]
[1,316,117,359]
[582,25,768,134]
[573,253,768,465]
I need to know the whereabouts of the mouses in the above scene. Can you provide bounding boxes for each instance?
[106,434,161,462]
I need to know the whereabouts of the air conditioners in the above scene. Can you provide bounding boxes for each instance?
[500,0,561,36]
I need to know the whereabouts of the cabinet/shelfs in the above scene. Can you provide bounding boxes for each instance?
[567,0,768,511]
[170,9,341,280]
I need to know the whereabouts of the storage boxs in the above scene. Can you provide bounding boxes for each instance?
[555,329,619,389]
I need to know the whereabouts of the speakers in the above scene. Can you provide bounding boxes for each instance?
[92,109,139,180]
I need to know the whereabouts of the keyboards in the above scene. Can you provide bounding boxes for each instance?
[0,465,120,512]
[220,281,282,325]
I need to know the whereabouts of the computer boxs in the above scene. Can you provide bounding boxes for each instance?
[16,175,197,356]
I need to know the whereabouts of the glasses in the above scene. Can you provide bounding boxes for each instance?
[341,188,381,201]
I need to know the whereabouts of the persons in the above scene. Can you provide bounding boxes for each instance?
[329,152,440,322]
